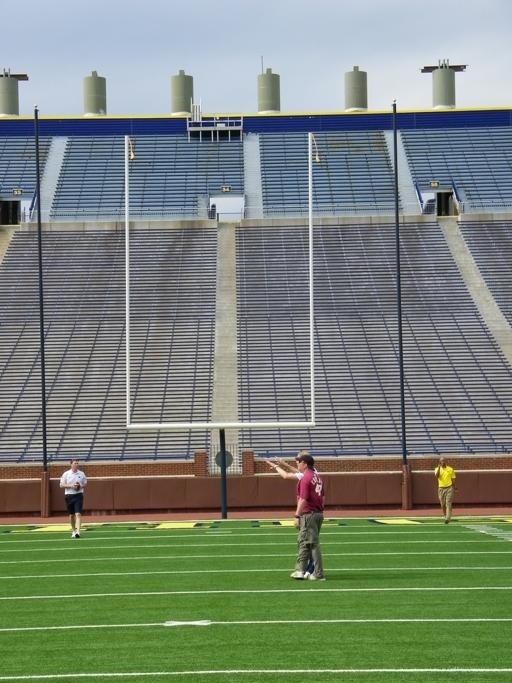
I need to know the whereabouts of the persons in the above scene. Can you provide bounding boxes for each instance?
[435,456,458,524]
[59,459,87,538]
[264,451,326,581]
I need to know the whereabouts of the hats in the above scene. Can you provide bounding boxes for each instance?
[295,453,316,471]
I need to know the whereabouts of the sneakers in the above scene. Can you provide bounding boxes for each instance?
[289,569,326,582]
[71,530,80,538]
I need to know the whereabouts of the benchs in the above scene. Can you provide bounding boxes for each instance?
[0,125,512,463]
